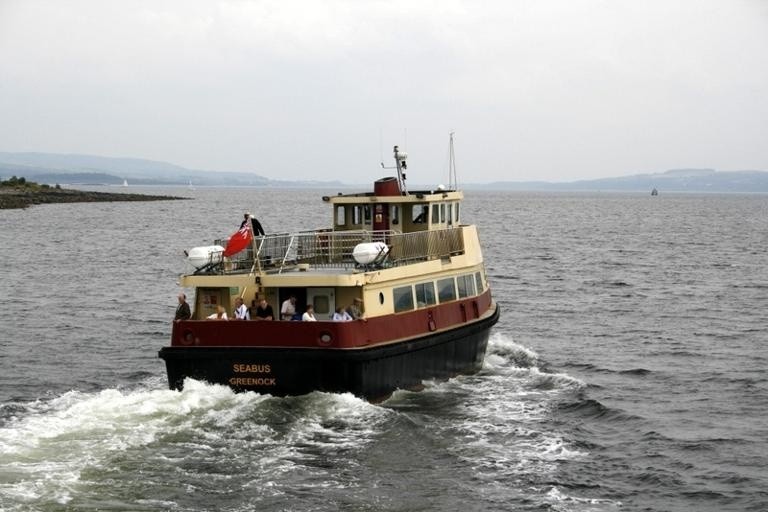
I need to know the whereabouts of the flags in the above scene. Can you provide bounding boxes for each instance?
[224,220,252,258]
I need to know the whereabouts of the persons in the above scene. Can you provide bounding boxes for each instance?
[173,293,192,323]
[254,298,276,322]
[229,298,250,321]
[413,206,428,223]
[301,303,317,321]
[281,295,297,320]
[206,302,228,320]
[333,304,355,322]
[345,297,367,324]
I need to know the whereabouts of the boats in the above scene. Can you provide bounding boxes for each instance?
[157,134,501,404]
